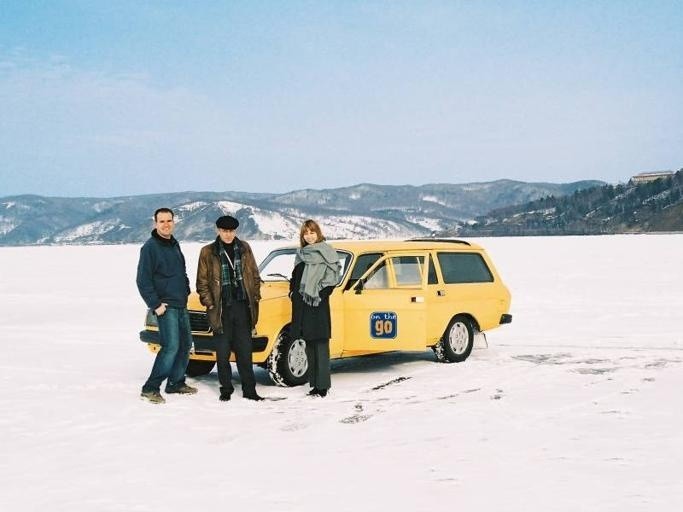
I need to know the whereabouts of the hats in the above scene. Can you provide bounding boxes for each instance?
[215,215,240,229]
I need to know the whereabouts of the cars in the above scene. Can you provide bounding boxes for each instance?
[137,235,513,389]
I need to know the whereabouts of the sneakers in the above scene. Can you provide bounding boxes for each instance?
[139,390,165,405]
[218,391,232,402]
[307,386,329,399]
[165,384,199,395]
[243,392,266,401]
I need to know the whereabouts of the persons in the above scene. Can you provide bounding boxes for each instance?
[135,207,199,406]
[196,215,266,402]
[286,219,341,399]
